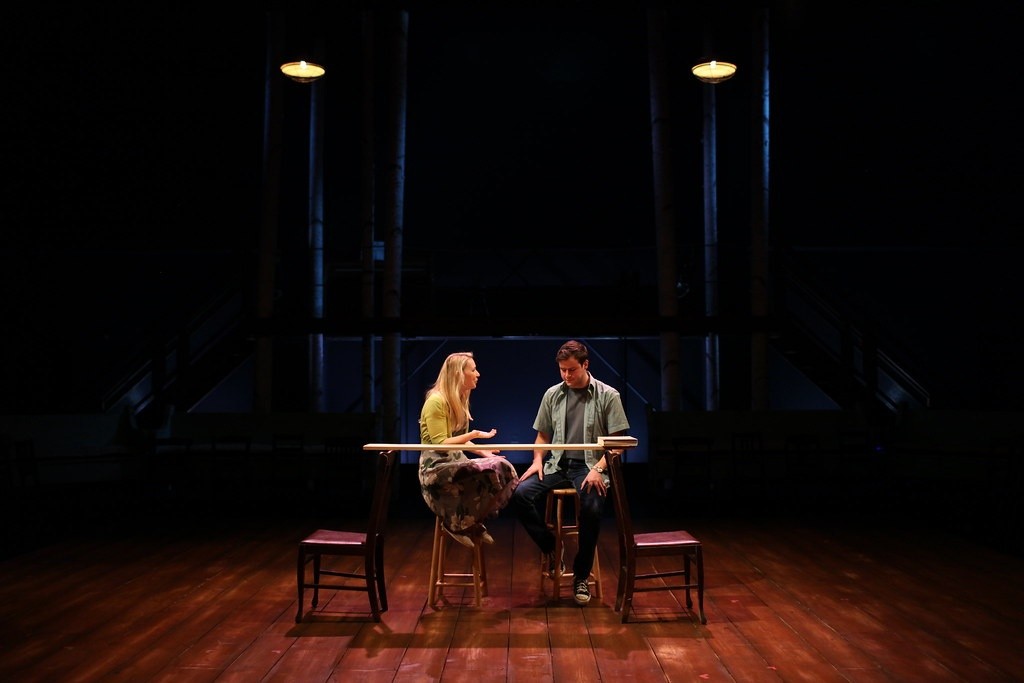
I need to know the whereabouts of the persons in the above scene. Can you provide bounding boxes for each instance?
[511,340,631,605]
[418,352,518,548]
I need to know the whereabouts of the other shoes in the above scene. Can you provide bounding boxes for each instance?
[440,522,475,547]
[482,533,495,544]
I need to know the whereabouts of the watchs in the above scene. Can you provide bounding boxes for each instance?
[591,466,604,474]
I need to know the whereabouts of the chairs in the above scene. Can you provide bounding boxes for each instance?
[295,450,396,624]
[603,450,708,625]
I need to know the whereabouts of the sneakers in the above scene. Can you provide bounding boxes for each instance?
[548,541,566,578]
[573,575,591,604]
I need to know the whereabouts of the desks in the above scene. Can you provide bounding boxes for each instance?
[361,443,636,451]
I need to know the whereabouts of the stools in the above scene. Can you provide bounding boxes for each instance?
[429,512,488,608]
[539,487,602,601]
